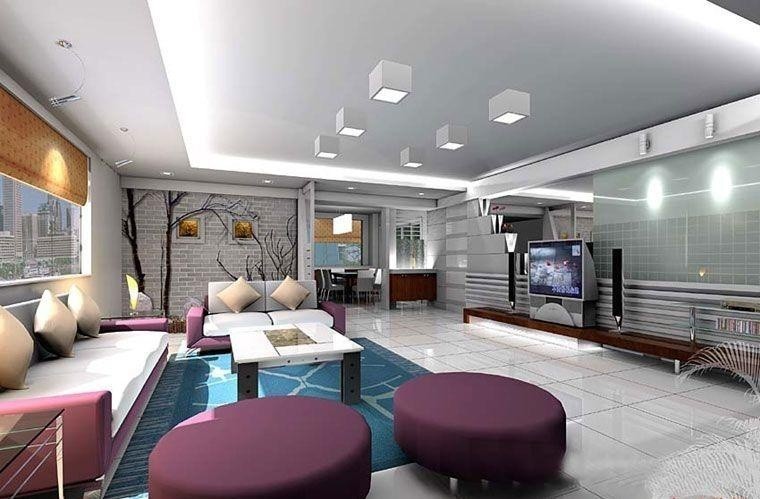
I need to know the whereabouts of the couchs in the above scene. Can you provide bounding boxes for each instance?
[186,280,346,356]
[0,288,169,498]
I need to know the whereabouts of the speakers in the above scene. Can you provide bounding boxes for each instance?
[507,252,517,314]
[609,248,626,333]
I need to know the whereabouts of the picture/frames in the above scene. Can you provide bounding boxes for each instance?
[170,212,207,246]
[226,214,260,246]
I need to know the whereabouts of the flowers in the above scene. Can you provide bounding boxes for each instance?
[491,205,506,214]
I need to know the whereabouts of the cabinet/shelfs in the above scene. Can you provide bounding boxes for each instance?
[390,273,436,301]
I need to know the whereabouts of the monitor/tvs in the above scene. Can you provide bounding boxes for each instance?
[528,239,598,328]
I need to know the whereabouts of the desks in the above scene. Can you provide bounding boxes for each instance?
[461,304,760,389]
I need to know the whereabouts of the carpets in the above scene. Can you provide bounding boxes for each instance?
[39,338,433,499]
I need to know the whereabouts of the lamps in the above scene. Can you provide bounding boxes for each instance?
[488,88,531,126]
[435,121,468,153]
[335,105,367,138]
[368,58,412,105]
[314,135,340,160]
[399,146,424,169]
[332,212,353,235]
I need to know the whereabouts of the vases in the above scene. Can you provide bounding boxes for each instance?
[490,214,505,236]
[477,195,491,217]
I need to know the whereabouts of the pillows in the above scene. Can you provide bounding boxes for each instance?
[269,275,310,311]
[67,284,101,338]
[215,276,262,314]
[34,289,77,358]
[0,305,34,390]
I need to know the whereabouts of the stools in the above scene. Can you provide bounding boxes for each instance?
[148,396,372,499]
[393,372,566,484]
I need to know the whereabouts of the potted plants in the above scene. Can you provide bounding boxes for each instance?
[502,223,518,252]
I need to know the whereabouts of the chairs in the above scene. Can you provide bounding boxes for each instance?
[315,268,326,301]
[352,270,376,306]
[322,269,349,303]
[368,268,382,304]
